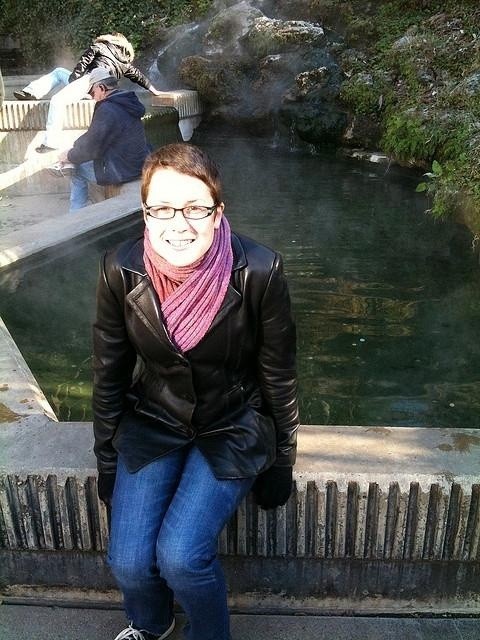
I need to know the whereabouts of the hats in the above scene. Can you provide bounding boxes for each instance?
[87,68,115,95]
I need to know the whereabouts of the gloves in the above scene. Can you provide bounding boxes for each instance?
[98,473,116,504]
[253,466,293,510]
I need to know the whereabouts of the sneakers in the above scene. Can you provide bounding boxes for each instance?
[114,616,176,639]
[35,144,54,153]
[42,162,77,178]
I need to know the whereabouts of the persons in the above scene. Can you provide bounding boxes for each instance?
[41,65,150,210]
[13,29,169,153]
[90,143,300,640]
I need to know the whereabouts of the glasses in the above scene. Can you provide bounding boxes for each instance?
[13,91,38,99]
[142,201,219,220]
